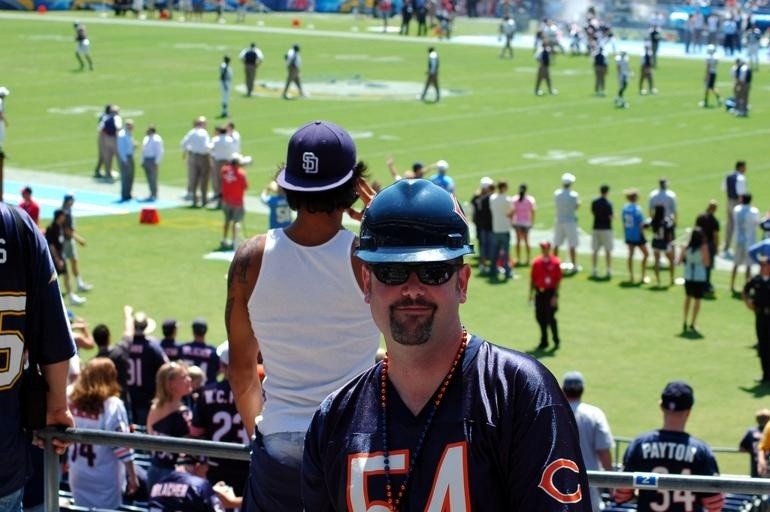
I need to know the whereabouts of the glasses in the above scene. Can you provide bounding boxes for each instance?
[361,261,469,285]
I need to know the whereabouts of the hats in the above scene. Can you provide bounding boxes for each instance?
[274,123,358,193]
[659,380,696,412]
[354,178,475,264]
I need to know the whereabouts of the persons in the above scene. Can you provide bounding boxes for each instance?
[0,86,770,512]
[0,1,770,118]
